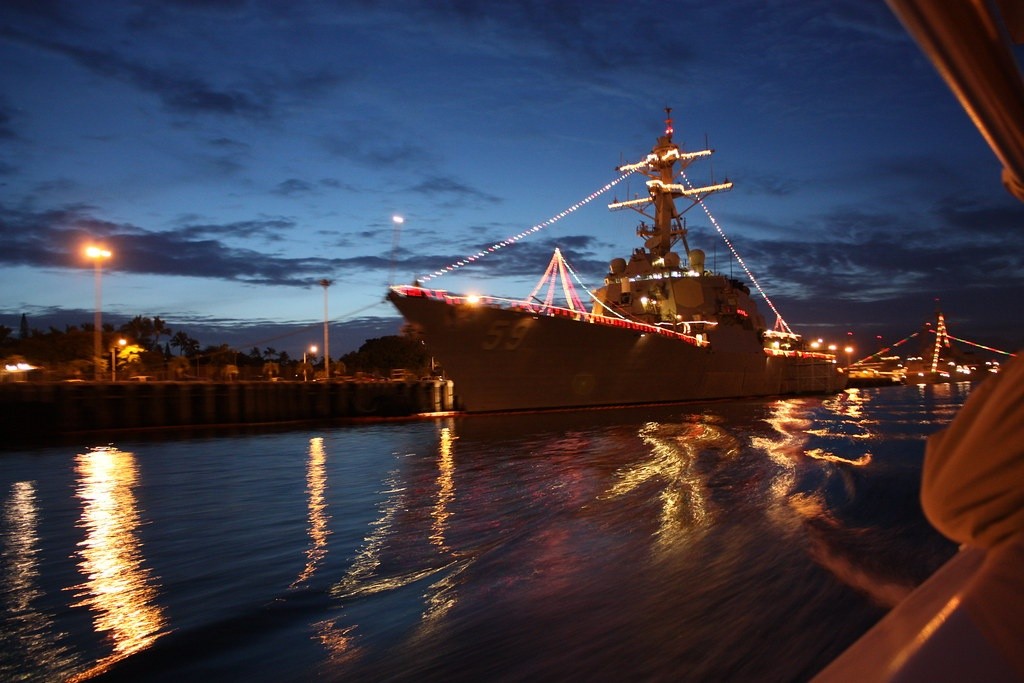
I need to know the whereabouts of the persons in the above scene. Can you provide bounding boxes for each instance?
[921,353,1024,676]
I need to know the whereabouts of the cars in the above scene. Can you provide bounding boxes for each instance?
[391,368,407,380]
[130,376,156,381]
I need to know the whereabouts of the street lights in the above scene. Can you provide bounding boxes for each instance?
[318,277,334,379]
[303,346,320,381]
[86,244,111,383]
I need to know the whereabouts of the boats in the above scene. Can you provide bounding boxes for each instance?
[386,103,909,421]
